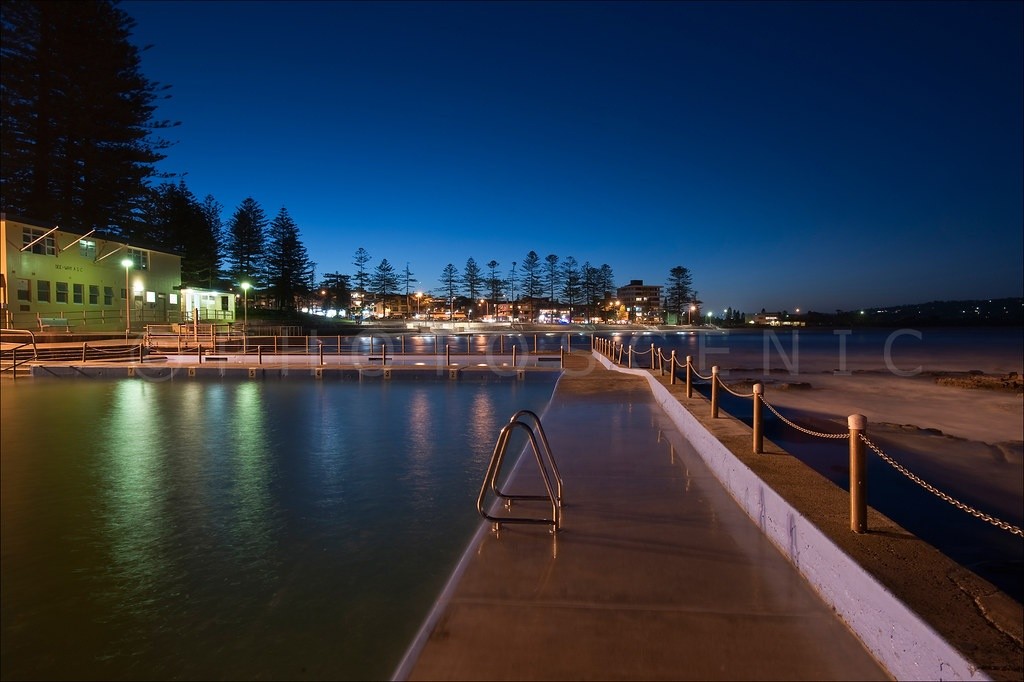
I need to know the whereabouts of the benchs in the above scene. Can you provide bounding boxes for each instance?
[38,317,76,333]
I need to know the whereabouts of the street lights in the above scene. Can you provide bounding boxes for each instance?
[241,281,250,325]
[687,306,695,325]
[708,312,712,324]
[417,292,422,314]
[122,259,133,331]
